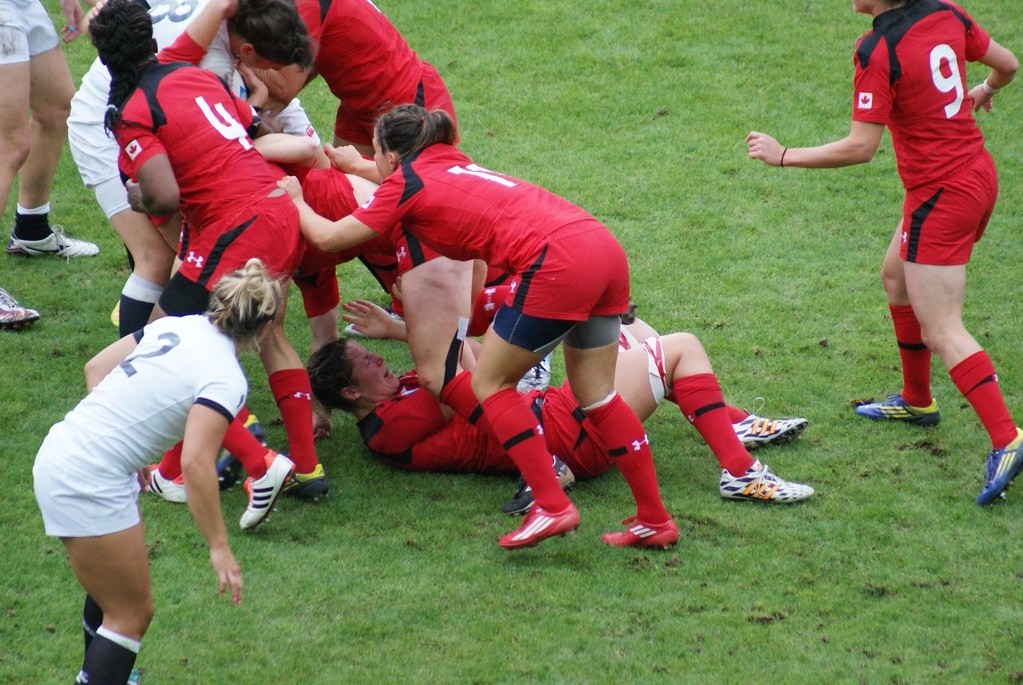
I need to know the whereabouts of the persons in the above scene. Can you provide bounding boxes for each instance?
[283,102,679,550]
[0,0,100,332]
[32,256,281,685]
[68,0,574,531]
[302,297,814,506]
[747,0,1023,505]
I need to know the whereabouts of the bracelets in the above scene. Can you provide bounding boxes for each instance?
[983,78,1000,94]
[781,148,787,167]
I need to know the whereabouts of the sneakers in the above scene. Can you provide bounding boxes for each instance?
[502,455,576,514]
[720,460,815,504]
[240,449,296,531]
[278,464,328,501]
[0,287,39,329]
[731,397,808,449]
[602,516,679,549]
[530,354,552,391]
[6,224,99,263]
[217,415,264,490]
[856,393,941,426]
[143,464,187,503]
[499,505,581,549]
[977,427,1023,505]
